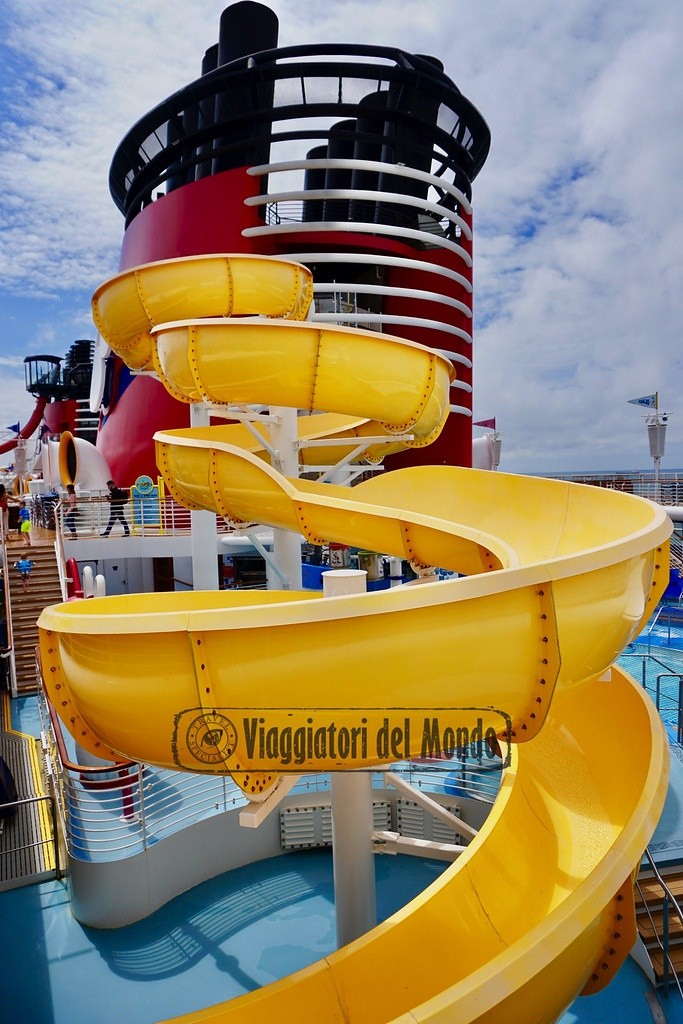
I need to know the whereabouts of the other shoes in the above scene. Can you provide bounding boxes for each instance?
[100,534,108,538]
[25,542,30,546]
[121,535,130,537]
[68,535,78,540]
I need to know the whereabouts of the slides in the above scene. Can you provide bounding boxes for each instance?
[30,253,676,1024]
[0,396,47,454]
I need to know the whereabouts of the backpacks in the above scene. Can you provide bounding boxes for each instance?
[112,490,128,504]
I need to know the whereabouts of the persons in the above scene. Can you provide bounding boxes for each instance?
[100,480,130,537]
[17,501,31,546]
[65,484,78,540]
[14,553,36,593]
[0,484,21,539]
